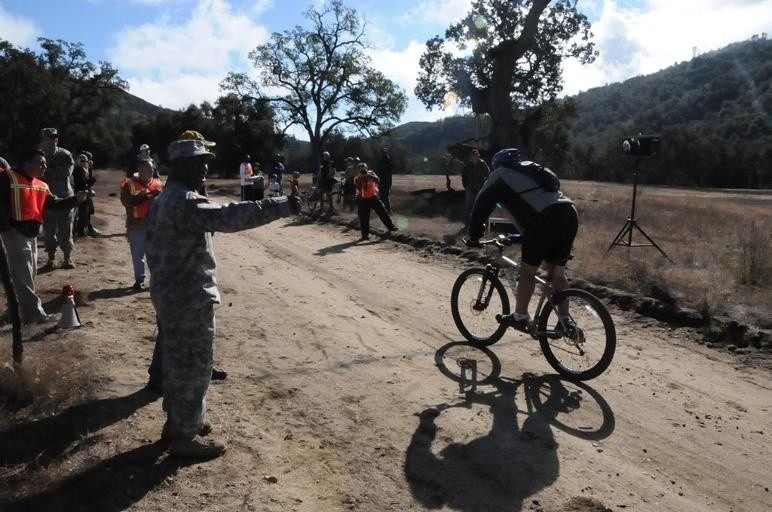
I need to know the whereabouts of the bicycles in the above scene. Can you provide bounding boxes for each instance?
[450,231,617,381]
[266,173,359,212]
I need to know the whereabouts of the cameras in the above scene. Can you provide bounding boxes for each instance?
[359,168,367,175]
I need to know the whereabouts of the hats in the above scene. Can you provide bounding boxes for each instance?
[41,127,60,138]
[180,130,215,147]
[167,140,216,162]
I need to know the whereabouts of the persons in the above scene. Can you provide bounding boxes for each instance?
[238,146,399,237]
[2,128,96,323]
[461,148,491,228]
[120,129,303,459]
[466,147,579,334]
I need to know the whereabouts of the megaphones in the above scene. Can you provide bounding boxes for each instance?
[56,285,83,329]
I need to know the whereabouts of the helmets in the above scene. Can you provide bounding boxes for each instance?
[490,148,521,170]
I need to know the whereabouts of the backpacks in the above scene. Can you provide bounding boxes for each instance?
[512,160,560,193]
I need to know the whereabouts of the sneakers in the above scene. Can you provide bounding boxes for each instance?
[496,314,529,330]
[161,418,213,441]
[172,434,227,459]
[553,318,569,330]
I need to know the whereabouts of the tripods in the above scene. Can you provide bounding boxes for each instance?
[602,155,676,264]
